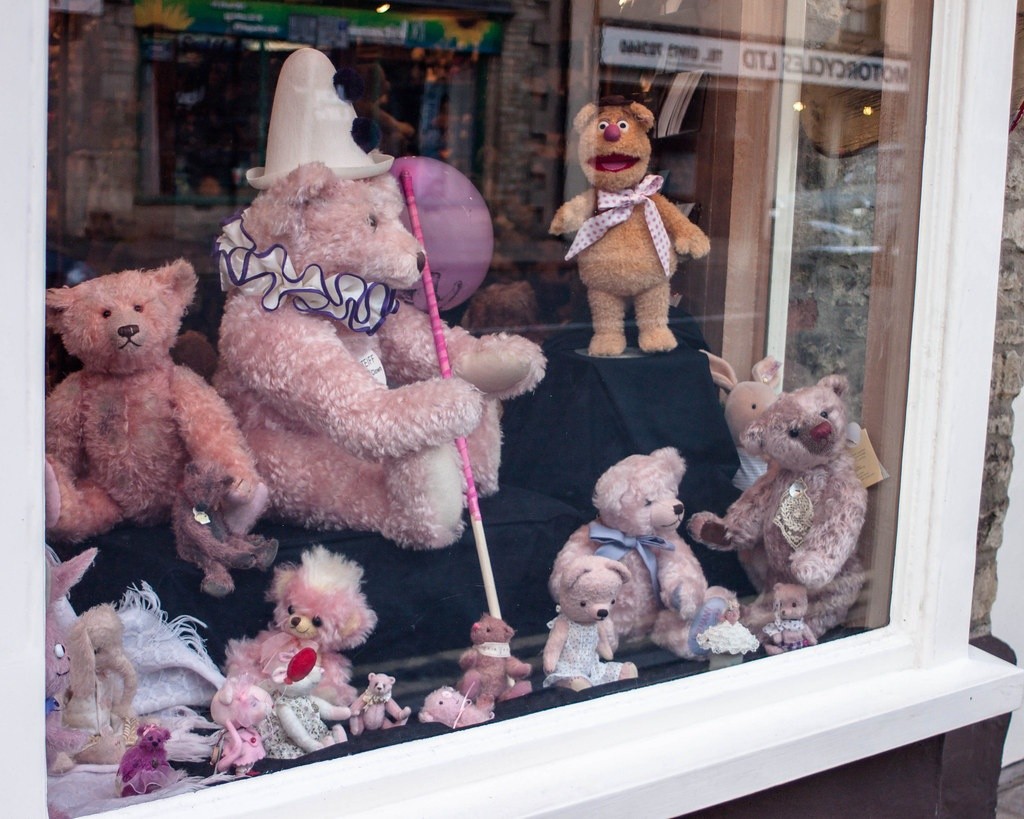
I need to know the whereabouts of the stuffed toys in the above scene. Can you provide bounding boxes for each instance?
[457,613,532,706]
[353,58,412,151]
[763,580,817,654]
[170,462,280,595]
[546,101,713,355]
[418,683,493,729]
[45,542,410,816]
[45,258,270,539]
[208,47,548,549]
[542,556,639,694]
[556,446,759,670]
[172,328,219,379]
[691,346,868,646]
[174,41,255,206]
[462,281,537,338]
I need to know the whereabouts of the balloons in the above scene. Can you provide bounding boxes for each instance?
[389,155,495,314]
